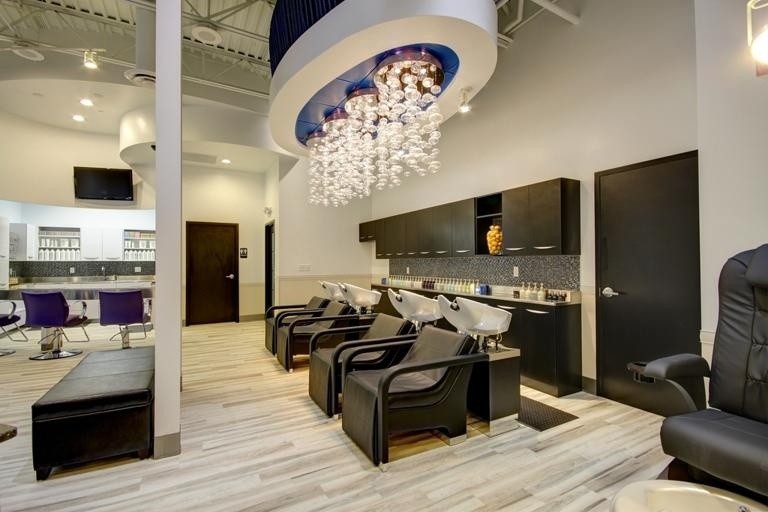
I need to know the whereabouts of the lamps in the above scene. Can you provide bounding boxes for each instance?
[743,18,767,80]
[457,85,473,115]
[81,49,100,70]
[297,50,447,207]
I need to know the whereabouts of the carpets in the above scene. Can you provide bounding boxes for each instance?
[515,394,578,434]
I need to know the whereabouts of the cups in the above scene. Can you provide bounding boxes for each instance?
[125,241,131,248]
[40,239,50,247]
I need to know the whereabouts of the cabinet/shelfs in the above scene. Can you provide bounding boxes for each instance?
[371,280,582,399]
[375,176,580,259]
[123,228,155,261]
[358,219,376,244]
[37,226,80,260]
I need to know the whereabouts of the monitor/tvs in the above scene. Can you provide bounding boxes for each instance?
[73,166,133,202]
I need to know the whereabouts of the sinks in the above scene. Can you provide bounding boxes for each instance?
[386,287,444,324]
[437,294,513,338]
[318,280,346,302]
[337,282,383,308]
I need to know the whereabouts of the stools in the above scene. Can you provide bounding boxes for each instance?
[0,299,28,358]
[612,478,765,512]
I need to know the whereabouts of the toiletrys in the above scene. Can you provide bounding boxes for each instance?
[524,281,531,299]
[519,281,525,299]
[537,282,547,301]
[531,281,537,300]
[388,274,481,295]
[37,238,80,261]
[123,231,156,261]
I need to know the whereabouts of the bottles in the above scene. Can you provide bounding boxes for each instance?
[39,249,80,261]
[519,281,544,300]
[388,273,481,295]
[125,250,155,261]
[486,225,504,255]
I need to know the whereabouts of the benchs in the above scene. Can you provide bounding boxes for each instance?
[30,344,156,484]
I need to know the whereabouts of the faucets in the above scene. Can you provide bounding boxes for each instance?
[100,265,107,281]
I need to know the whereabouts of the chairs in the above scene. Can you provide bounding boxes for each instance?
[20,290,91,361]
[263,295,491,469]
[98,287,150,348]
[634,243,766,504]
